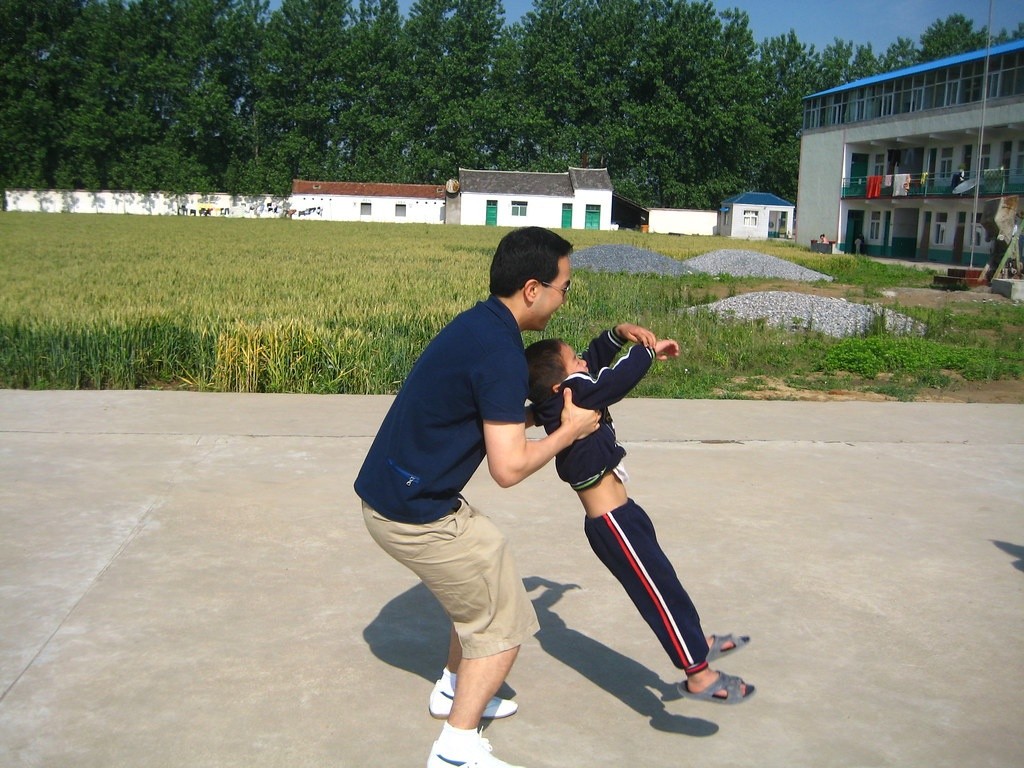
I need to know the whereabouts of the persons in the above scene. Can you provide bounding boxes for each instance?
[820,234,828,244]
[353,226,603,766]
[858,232,864,252]
[524,324,757,703]
[855,239,861,254]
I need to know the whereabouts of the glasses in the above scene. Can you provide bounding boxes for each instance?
[539,281,570,299]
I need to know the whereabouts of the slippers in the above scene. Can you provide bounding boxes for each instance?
[705,634,750,663]
[677,670,756,704]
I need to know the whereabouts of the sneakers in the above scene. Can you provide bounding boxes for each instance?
[429,680,518,720]
[427,741,526,768]
